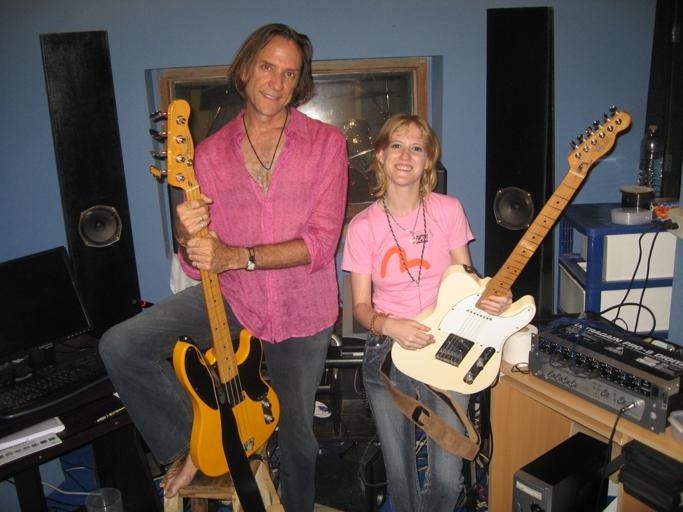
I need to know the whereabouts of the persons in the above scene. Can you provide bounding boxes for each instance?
[339,112,512,511]
[97,24,350,510]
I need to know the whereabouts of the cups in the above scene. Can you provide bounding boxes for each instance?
[84,487,122,512]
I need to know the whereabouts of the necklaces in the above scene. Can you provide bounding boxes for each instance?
[242,106,287,185]
[382,191,425,285]
[387,201,420,243]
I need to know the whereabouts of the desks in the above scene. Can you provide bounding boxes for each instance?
[0,389,163,512]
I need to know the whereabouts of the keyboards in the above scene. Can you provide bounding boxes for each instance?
[1,351,110,421]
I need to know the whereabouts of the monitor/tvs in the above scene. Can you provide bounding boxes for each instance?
[0,246,97,369]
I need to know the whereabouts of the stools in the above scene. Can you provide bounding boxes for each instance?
[163,434,284,512]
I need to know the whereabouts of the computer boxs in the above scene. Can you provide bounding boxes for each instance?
[511,432,611,512]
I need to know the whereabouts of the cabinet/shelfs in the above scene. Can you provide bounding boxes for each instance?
[483,375,683,512]
[551,197,676,338]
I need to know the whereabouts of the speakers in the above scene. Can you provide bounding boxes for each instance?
[40,30,142,337]
[485,8,556,324]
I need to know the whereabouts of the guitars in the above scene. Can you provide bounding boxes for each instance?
[148,99,280,478]
[390,103,634,395]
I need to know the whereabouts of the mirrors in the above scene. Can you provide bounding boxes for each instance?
[143,57,427,261]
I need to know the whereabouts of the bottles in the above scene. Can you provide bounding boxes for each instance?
[637,124,666,200]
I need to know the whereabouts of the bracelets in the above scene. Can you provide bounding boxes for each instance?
[369,313,384,335]
[245,246,255,272]
